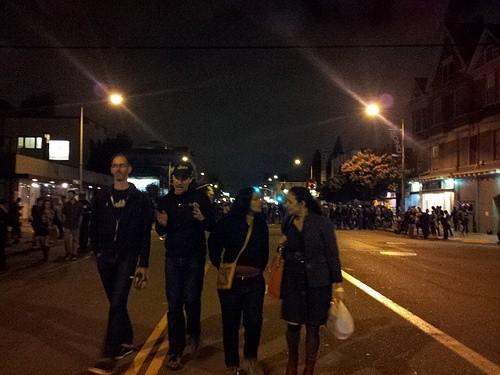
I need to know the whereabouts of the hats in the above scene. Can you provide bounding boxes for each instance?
[170,161,195,181]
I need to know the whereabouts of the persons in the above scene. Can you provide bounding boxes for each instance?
[216,197,469,239]
[208,187,270,375]
[279,186,342,374]
[0,189,95,272]
[155,161,216,371]
[87,153,152,375]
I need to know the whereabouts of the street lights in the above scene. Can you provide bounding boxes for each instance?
[365,103,405,215]
[295,159,313,200]
[79,90,124,194]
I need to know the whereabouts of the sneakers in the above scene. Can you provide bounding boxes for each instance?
[88,357,120,375]
[114,344,133,360]
[189,335,200,355]
[168,352,183,368]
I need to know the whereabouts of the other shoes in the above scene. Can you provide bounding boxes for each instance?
[65,253,77,260]
[228,367,238,375]
[244,358,256,375]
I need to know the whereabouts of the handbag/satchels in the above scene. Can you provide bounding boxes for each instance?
[267,254,286,300]
[216,262,236,289]
[326,287,354,340]
